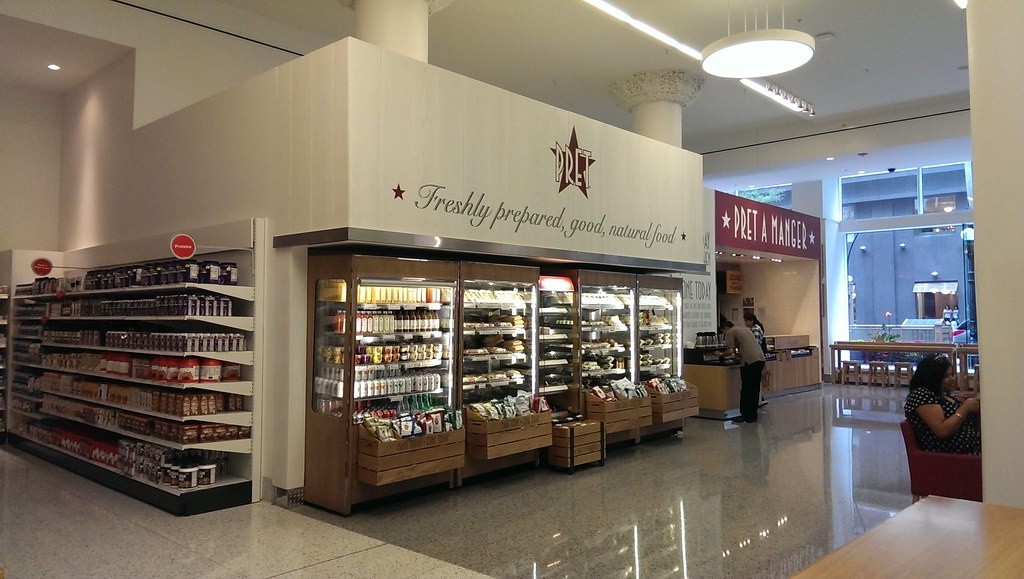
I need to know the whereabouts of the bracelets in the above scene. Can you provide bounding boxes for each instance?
[720,352,723,356]
[955,412,965,422]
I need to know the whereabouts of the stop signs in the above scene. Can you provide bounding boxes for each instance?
[31,258,53,277]
[170,234,197,260]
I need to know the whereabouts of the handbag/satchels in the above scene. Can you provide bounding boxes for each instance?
[760,337,767,353]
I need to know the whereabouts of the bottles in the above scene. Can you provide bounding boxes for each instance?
[84,259,238,290]
[397,394,433,412]
[316,279,453,304]
[117,439,227,486]
[99,299,156,316]
[331,310,440,334]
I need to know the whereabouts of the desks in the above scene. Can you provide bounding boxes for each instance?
[957,344,979,392]
[789,495,1024,579]
[829,341,957,392]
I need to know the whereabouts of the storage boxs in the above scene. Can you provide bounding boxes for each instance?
[543,421,606,474]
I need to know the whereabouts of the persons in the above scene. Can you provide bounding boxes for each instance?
[951,305,959,327]
[743,312,768,408]
[904,353,981,456]
[713,321,765,423]
[942,305,953,322]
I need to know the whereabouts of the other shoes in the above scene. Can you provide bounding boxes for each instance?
[732,416,752,423]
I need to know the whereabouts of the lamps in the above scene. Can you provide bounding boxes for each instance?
[700,0,815,78]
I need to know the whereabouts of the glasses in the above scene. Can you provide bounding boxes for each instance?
[934,354,947,363]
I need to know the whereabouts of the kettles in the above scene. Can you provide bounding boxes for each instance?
[696,332,718,345]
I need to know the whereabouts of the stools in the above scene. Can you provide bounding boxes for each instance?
[840,360,864,386]
[889,362,916,389]
[973,364,979,392]
[867,361,890,388]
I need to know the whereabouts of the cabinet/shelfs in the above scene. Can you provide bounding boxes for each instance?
[0,216,267,517]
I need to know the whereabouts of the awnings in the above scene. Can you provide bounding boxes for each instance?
[913,282,958,293]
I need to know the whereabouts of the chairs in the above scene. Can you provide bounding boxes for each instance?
[899,420,983,506]
